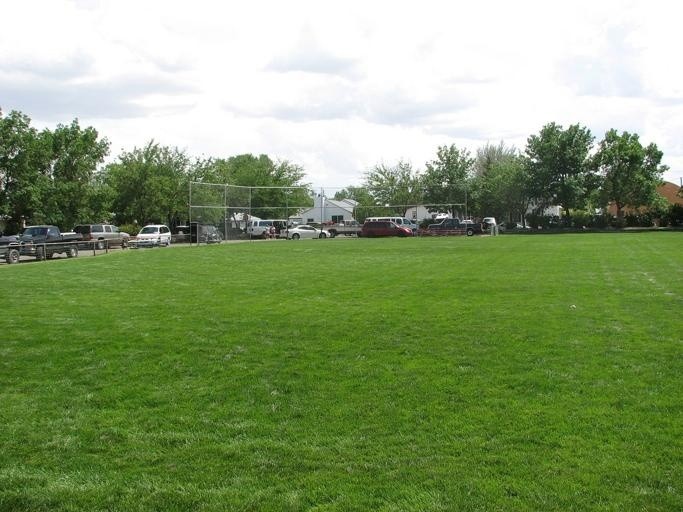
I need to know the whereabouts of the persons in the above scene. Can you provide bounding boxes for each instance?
[265,223,270,240]
[270,225,275,240]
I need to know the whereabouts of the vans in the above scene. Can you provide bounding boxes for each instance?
[246,219,293,239]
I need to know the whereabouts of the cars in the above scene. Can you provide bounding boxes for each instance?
[498,223,523,229]
[322,217,496,236]
[279,224,332,239]
[0,222,170,263]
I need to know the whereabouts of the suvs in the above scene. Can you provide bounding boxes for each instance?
[191,224,224,243]
[173,225,190,241]
